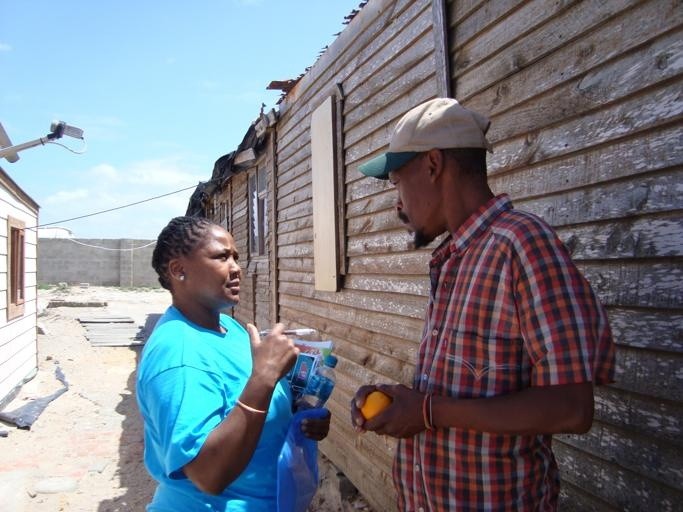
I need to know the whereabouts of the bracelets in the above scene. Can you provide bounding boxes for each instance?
[422,393,431,429]
[429,393,432,427]
[237,400,267,414]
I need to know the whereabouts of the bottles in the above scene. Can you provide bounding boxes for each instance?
[298,355,338,413]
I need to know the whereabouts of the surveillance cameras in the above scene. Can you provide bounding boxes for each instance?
[49,119,85,140]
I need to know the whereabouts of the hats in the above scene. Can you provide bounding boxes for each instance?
[357,97,493,179]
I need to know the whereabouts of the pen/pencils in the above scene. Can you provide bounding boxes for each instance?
[259,328,317,337]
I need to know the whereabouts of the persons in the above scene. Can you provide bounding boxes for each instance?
[350,97,616,512]
[135,216,332,512]
